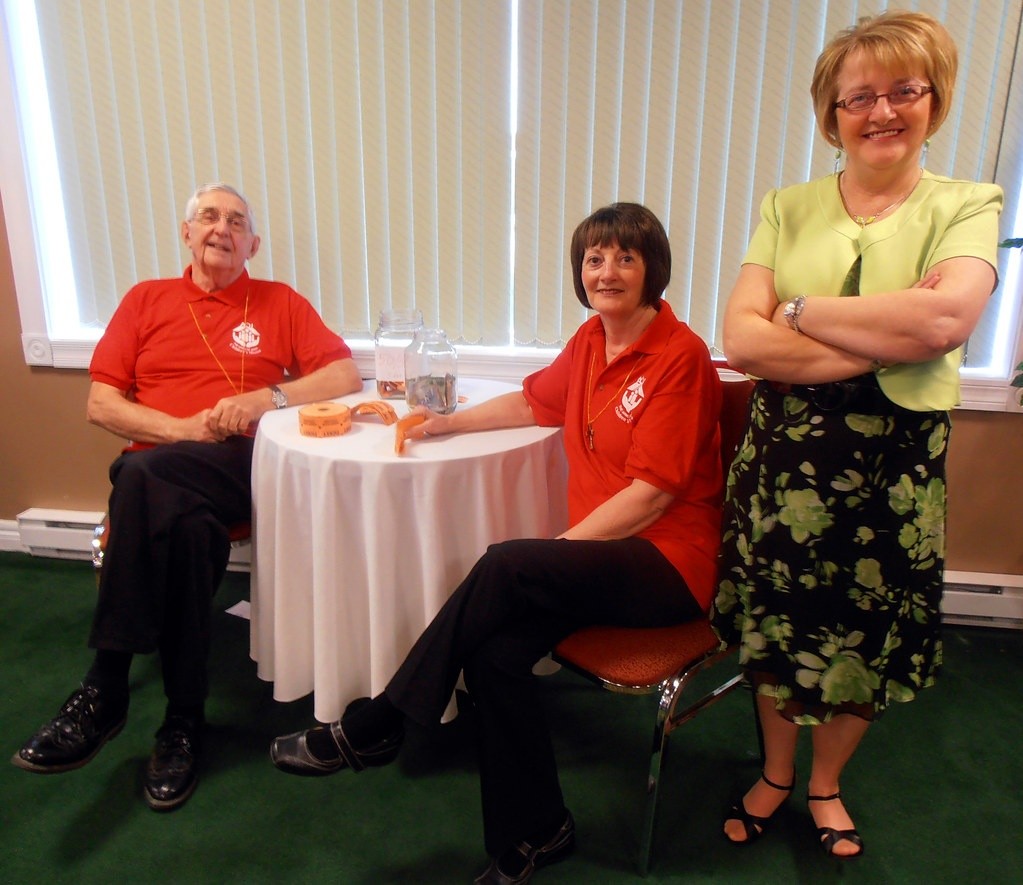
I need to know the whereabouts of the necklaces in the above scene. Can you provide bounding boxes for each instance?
[188,293,249,395]
[586,352,644,456]
[838,171,923,227]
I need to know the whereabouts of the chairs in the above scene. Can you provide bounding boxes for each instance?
[543,361,761,880]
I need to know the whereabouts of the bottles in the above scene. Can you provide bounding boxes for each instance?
[404,327,457,416]
[374,308,424,399]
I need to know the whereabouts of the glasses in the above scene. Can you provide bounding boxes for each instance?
[831,84,936,112]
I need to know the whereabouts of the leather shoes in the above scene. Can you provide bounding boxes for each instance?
[18,685,130,773]
[145,719,207,810]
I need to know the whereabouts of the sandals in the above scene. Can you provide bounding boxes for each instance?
[723,769,795,849]
[806,786,864,860]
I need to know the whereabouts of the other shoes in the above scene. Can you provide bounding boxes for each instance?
[269,695,405,777]
[474,808,576,885]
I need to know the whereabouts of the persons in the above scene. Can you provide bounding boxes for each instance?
[708,11,1003,863]
[269,201,726,885]
[13,181,363,813]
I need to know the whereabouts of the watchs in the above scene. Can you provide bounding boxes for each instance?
[267,383,288,410]
[783,295,803,329]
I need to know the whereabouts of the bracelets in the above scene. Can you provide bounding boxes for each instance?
[793,296,804,335]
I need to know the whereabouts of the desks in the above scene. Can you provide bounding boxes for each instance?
[249,376,570,734]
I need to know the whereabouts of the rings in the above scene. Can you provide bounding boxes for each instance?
[423,431,431,437]
